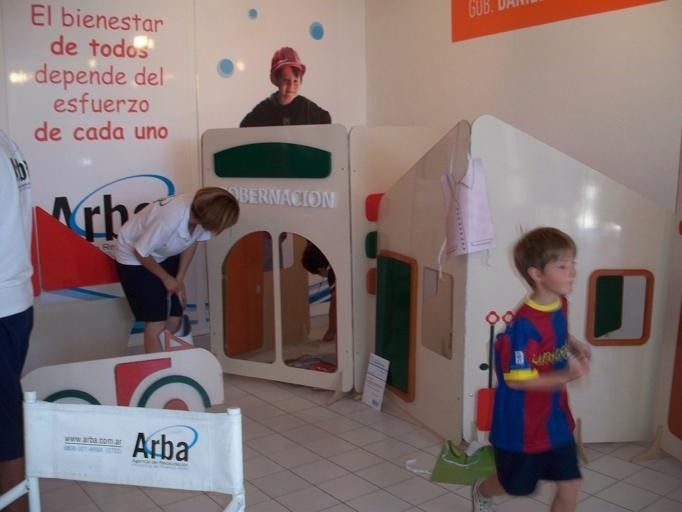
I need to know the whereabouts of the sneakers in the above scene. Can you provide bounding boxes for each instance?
[473,479,492,512]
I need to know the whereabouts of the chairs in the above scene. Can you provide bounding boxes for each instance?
[0,392,245,512]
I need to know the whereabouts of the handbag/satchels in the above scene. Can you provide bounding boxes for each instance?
[158,292,193,350]
[431,440,498,484]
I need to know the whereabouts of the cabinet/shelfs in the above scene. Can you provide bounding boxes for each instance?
[376,113,682,460]
[201,125,419,395]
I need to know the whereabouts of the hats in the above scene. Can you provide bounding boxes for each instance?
[271,48,306,85]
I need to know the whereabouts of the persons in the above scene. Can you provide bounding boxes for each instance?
[302,240,336,341]
[115,187,239,355]
[472,226,593,512]
[239,46,331,128]
[0,129,34,511]
